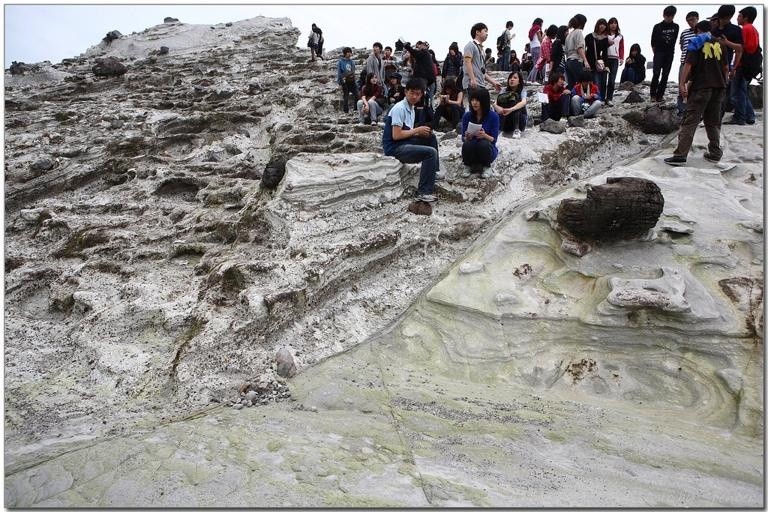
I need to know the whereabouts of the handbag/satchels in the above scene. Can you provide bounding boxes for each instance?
[741,47,762,81]
[456,71,463,90]
[595,59,605,72]
[497,30,509,53]
[497,91,517,120]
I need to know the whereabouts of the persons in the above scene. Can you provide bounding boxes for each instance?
[380,78,440,204]
[493,71,527,139]
[620,44,647,84]
[339,47,360,113]
[650,6,679,102]
[357,40,465,124]
[679,4,763,126]
[483,14,624,106]
[571,71,602,118]
[460,87,500,179]
[664,19,730,164]
[307,23,325,62]
[540,73,571,122]
[461,23,501,111]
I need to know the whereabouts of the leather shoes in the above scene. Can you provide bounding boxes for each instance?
[724,118,755,125]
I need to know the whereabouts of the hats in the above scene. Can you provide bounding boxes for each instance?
[388,72,402,80]
[707,13,718,20]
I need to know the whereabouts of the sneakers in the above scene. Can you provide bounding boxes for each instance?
[481,166,491,179]
[513,129,522,139]
[461,166,473,178]
[435,171,445,179]
[664,155,686,166]
[526,81,542,86]
[606,98,614,106]
[704,152,719,163]
[414,191,438,202]
